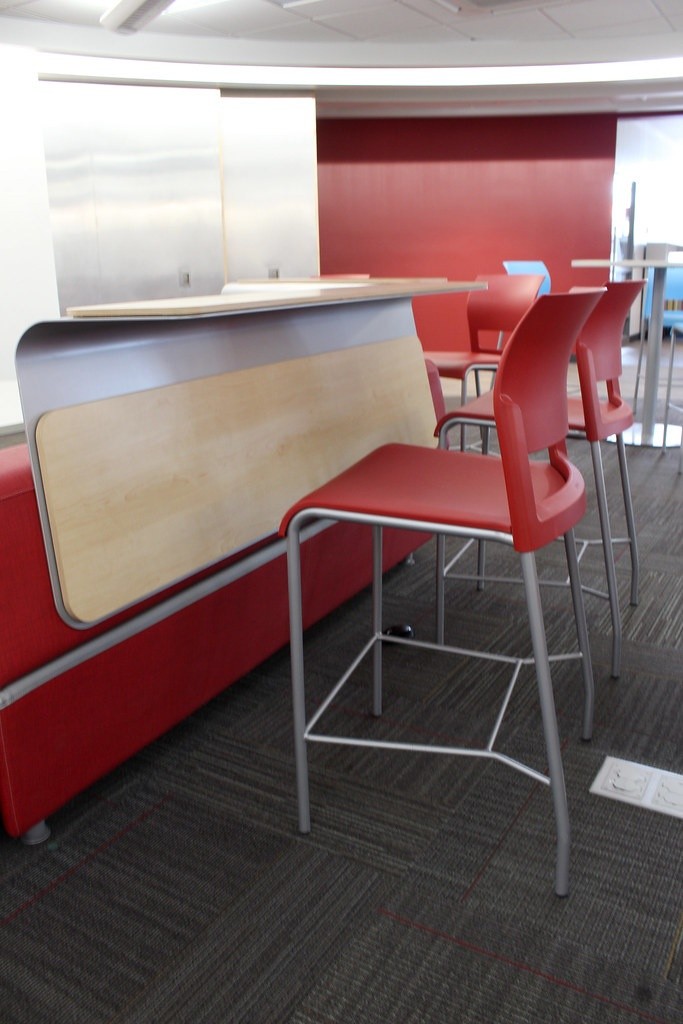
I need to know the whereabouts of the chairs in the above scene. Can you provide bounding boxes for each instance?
[277,286,610,898]
[632,264,683,477]
[423,261,648,744]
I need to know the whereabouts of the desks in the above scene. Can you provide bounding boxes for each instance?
[572,260,683,447]
[12,279,489,632]
